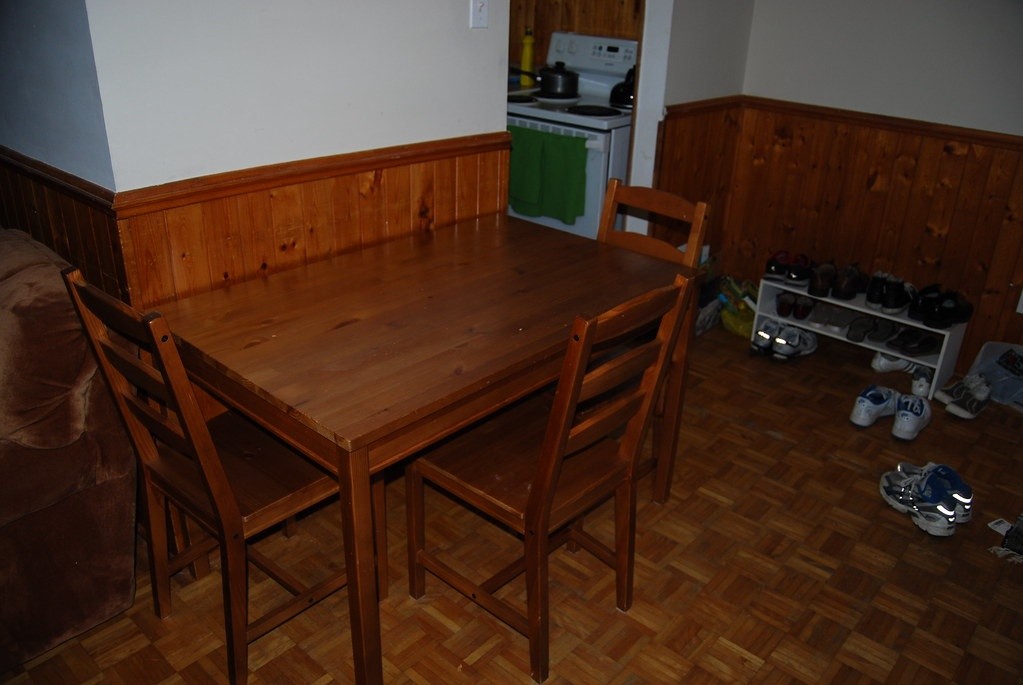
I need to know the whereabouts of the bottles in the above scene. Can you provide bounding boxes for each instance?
[520,28,534,87]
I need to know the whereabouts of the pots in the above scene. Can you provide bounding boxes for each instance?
[512,61,579,98]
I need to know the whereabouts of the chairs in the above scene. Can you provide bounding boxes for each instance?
[61,264,387,685]
[553,180,706,502]
[405,267,694,685]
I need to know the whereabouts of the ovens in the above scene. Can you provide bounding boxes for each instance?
[508,114,632,240]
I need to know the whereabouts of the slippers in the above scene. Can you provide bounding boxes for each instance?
[776,290,943,358]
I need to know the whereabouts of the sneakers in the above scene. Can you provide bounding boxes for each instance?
[911,367,933,398]
[870,349,919,374]
[934,369,993,418]
[849,383,901,427]
[764,250,973,329]
[751,318,782,351]
[892,394,931,441]
[898,462,972,524]
[772,326,818,360]
[879,473,956,538]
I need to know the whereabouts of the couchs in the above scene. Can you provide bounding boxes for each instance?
[0,226,140,672]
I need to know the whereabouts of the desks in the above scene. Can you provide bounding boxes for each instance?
[138,212,703,685]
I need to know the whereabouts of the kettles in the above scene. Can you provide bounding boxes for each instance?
[609,64,636,109]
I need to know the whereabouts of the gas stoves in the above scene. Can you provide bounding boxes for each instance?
[508,32,639,132]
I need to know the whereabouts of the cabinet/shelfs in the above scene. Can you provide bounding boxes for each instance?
[750,279,968,402]
